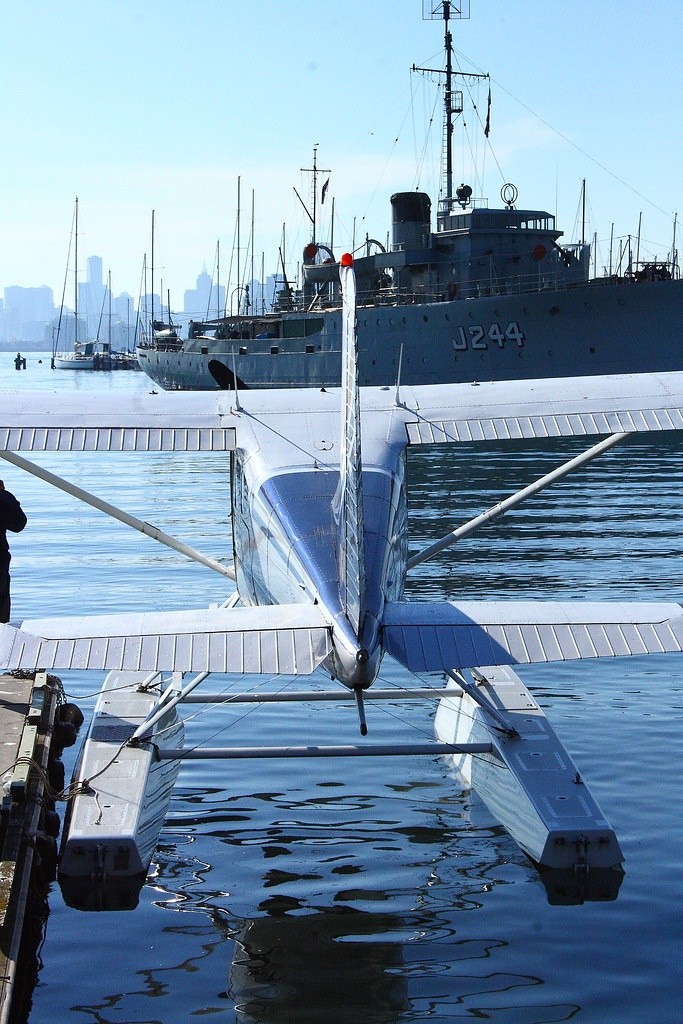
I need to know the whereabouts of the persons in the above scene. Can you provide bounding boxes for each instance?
[0,480,27,624]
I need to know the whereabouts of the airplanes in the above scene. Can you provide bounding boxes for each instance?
[1,252,682,880]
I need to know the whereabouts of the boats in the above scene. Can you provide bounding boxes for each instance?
[133,1,683,393]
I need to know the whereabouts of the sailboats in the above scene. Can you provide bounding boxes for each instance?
[50,171,170,373]
